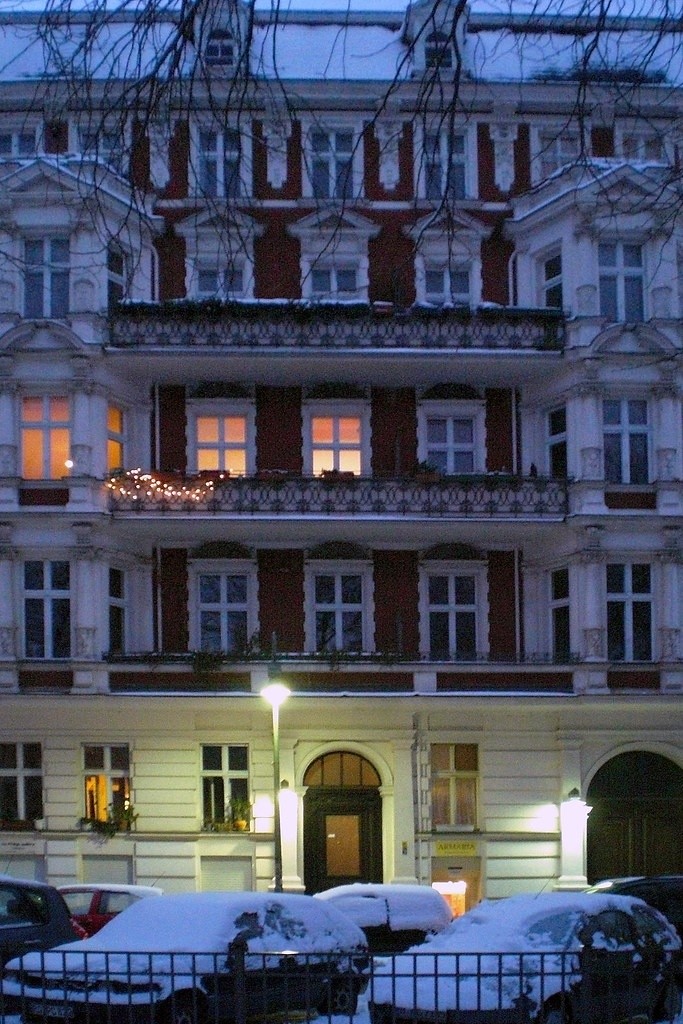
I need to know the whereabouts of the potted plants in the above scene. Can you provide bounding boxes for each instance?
[33,816,44,831]
[108,801,139,831]
[213,815,234,831]
[189,647,224,671]
[5,818,31,830]
[205,815,212,832]
[229,794,249,831]
[372,273,399,312]
[80,817,92,831]
[405,458,442,482]
[320,468,358,491]
[0,809,6,830]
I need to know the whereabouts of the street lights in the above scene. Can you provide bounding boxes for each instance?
[262,684,297,893]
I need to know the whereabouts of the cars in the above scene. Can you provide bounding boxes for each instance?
[367,893,683,1024]
[312,884,452,951]
[0,878,165,961]
[3,892,368,1024]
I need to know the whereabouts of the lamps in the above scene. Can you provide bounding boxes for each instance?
[569,788,580,799]
[280,780,290,789]
